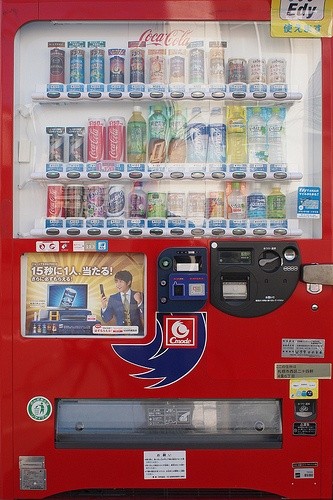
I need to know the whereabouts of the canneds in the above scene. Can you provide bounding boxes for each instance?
[226,58,286,83]
[144,192,225,218]
[47,185,126,218]
[49,48,225,85]
[86,116,126,160]
[49,134,84,163]
[59,288,76,307]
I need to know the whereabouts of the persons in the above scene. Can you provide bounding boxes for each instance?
[101,270,144,333]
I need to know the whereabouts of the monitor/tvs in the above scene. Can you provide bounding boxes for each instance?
[46,284,89,312]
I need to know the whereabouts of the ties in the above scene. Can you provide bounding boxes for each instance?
[122,293,131,327]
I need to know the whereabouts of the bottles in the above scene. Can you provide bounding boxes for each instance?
[168,105,186,163]
[209,107,226,162]
[226,105,247,163]
[227,183,246,219]
[128,182,147,219]
[248,107,267,164]
[267,107,286,164]
[247,182,266,219]
[126,106,147,163]
[149,105,167,163]
[187,107,207,163]
[266,183,286,219]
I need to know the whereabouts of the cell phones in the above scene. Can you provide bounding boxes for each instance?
[100,284,107,301]
